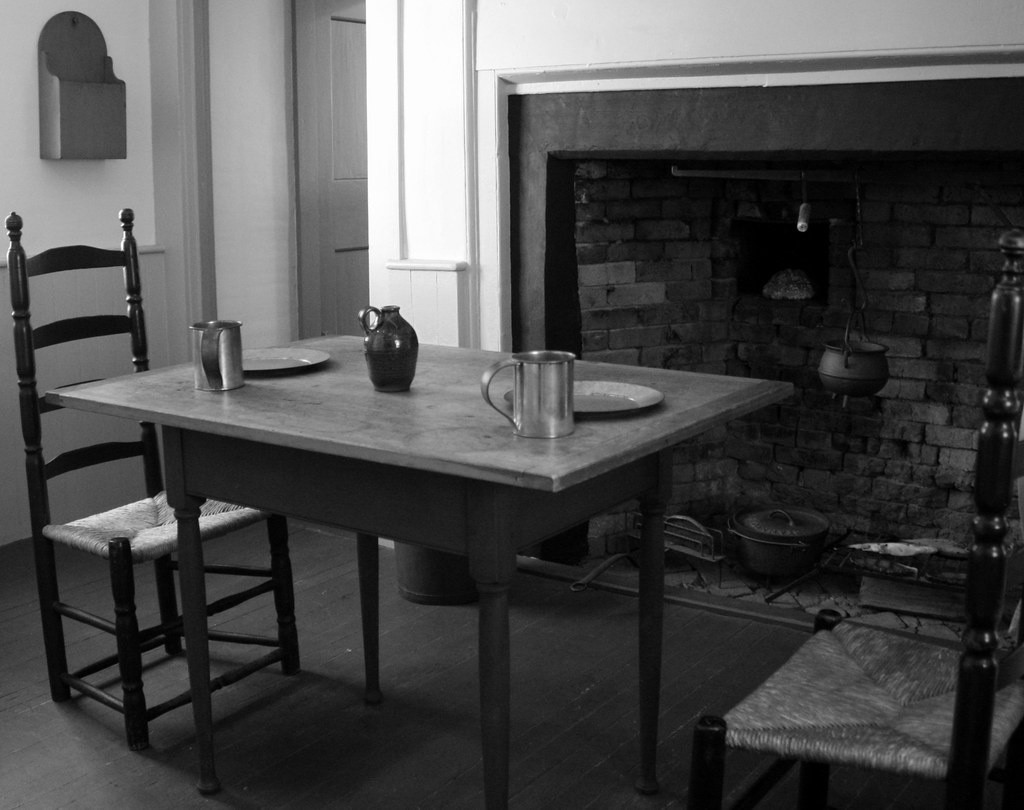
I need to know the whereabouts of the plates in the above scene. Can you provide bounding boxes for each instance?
[242,349,330,372]
[504,381,664,416]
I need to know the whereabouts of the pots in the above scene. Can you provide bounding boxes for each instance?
[727,504,831,576]
[817,340,890,407]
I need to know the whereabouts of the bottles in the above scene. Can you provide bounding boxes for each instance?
[357,306,419,391]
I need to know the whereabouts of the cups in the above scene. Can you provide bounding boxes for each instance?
[482,351,577,435]
[189,319,245,391]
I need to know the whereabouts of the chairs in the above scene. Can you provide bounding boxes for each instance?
[5,204,303,759]
[691,224,1024,810]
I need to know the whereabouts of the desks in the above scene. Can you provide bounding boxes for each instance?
[46,329,794,810]
